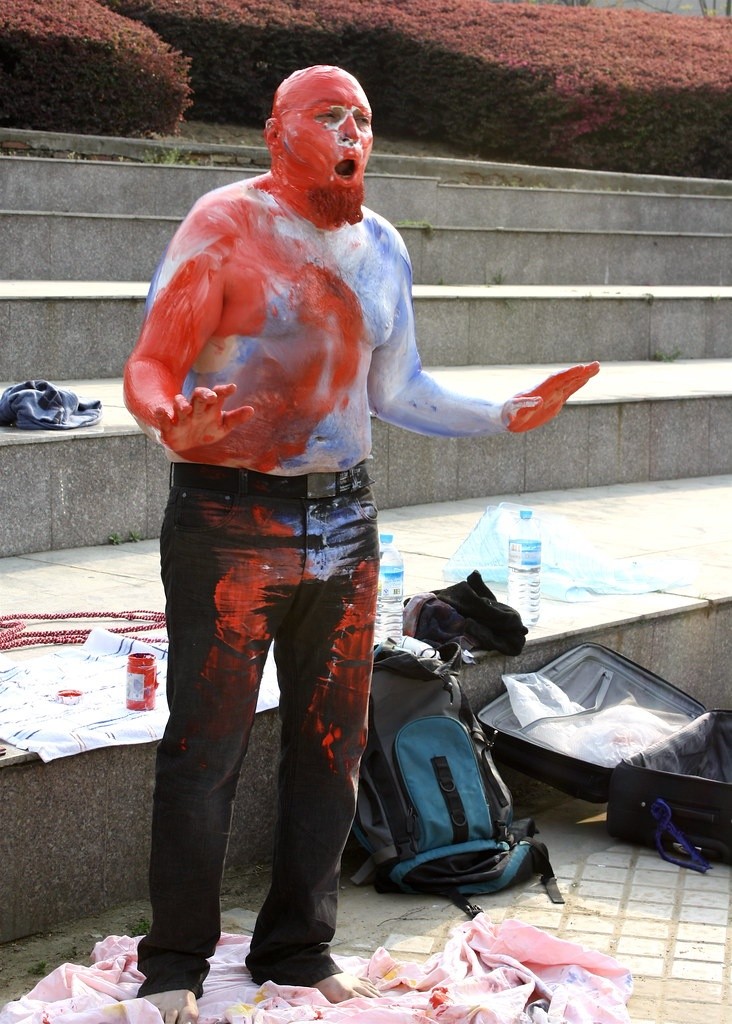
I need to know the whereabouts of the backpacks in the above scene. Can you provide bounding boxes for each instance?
[349,635,564,918]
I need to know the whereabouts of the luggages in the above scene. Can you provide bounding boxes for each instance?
[477,642,732,871]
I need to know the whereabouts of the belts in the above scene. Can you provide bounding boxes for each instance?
[170,462,367,501]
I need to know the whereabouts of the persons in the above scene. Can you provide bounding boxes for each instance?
[125,65,600,1017]
